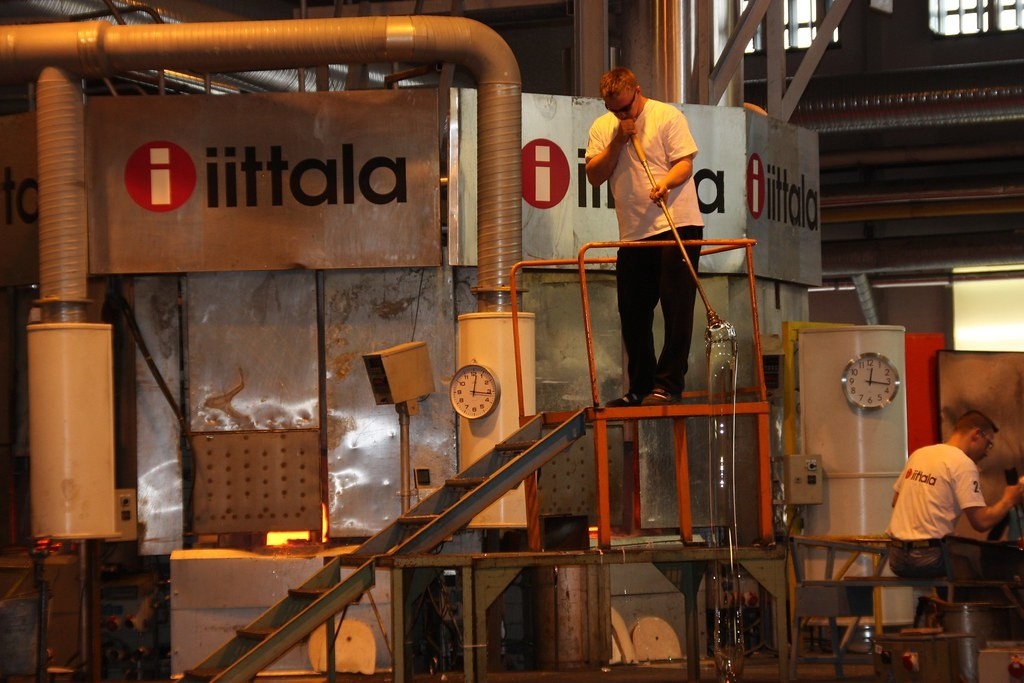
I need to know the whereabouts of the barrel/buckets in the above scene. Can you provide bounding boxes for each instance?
[523,563,612,670]
[941,606,1010,683]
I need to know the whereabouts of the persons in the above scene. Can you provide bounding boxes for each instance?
[885,410,1024,603]
[586,66,705,407]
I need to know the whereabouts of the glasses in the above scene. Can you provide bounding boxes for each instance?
[982,431,995,449]
[605,90,636,112]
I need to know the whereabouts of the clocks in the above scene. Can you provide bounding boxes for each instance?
[842,352,901,411]
[450,364,502,420]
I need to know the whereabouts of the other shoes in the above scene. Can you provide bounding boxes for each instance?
[605,391,642,407]
[640,388,678,405]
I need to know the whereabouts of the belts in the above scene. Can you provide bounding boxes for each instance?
[890,539,940,549]
